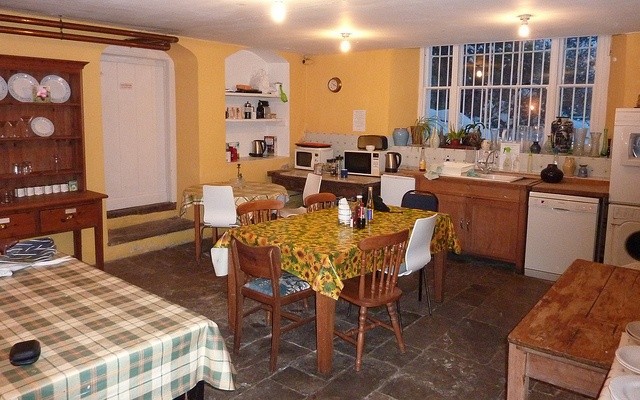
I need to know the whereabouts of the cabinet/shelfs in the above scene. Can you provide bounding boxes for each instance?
[0,54,90,200]
[417,179,519,274]
[225,92,281,122]
[267,168,381,201]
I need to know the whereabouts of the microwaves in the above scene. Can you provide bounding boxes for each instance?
[295,147,333,170]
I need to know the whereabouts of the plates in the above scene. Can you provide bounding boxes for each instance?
[0,76,8,102]
[625,320,640,343]
[40,75,71,103]
[8,73,40,103]
[615,345,640,374]
[31,117,55,137]
[609,375,640,400]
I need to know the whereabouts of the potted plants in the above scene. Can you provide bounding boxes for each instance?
[444,123,467,146]
[406,115,446,144]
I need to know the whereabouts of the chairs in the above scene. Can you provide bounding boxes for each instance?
[200,185,241,245]
[401,190,439,302]
[279,173,323,217]
[305,192,336,213]
[333,229,409,372]
[236,200,284,226]
[230,234,317,371]
[371,213,438,335]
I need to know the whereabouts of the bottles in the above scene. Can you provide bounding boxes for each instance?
[261,100,271,119]
[354,195,364,224]
[313,153,322,175]
[244,101,256,119]
[366,187,374,222]
[225,106,233,119]
[256,103,265,119]
[527,153,533,173]
[356,203,365,229]
[513,157,521,174]
[418,147,427,172]
[503,147,512,173]
[326,155,344,175]
[232,107,240,119]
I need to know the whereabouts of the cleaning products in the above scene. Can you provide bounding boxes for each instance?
[502,147,512,172]
[512,156,521,173]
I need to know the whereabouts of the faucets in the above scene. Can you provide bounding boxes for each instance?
[478,151,499,174]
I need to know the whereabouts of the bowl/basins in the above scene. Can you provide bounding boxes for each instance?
[366,145,375,152]
[338,198,353,225]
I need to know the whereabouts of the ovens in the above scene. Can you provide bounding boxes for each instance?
[343,149,386,178]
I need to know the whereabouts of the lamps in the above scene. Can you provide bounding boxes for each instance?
[340,33,351,52]
[476,64,483,77]
[517,14,532,37]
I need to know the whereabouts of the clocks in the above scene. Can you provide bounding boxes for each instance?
[328,77,342,93]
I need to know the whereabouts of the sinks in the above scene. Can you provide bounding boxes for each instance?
[466,174,523,183]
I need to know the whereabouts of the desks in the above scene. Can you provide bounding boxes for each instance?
[0,190,108,271]
[179,182,290,262]
[507,258,640,400]
[0,249,237,400]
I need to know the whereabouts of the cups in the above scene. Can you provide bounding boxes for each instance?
[19,164,29,175]
[23,161,33,173]
[341,169,347,178]
[10,162,22,175]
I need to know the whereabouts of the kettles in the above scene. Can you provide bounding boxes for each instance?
[384,152,401,173]
[249,140,266,157]
